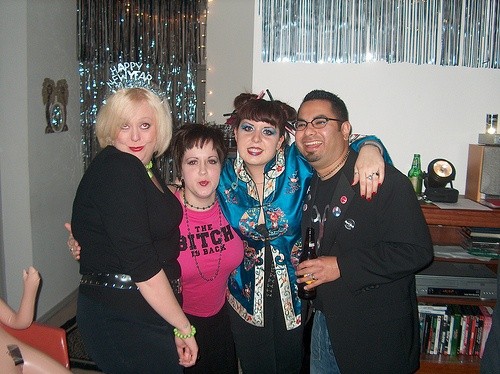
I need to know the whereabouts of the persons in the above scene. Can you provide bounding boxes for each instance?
[65,123,244,374]
[291,90,434,374]
[71,87,198,374]
[216,90,393,374]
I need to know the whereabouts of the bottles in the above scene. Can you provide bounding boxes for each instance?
[298,227,317,301]
[408,154,423,196]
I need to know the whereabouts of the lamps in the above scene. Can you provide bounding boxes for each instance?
[423,159,459,203]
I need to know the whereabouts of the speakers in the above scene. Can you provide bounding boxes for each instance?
[465,144,500,202]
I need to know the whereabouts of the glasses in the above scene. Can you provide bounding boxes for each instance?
[291,117,341,132]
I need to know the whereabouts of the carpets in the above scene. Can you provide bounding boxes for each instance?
[57,313,103,371]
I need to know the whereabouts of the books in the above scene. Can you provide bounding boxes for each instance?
[417,304,494,359]
[457,227,500,258]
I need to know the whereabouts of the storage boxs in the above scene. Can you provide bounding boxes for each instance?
[465,143,500,203]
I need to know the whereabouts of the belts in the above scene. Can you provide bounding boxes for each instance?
[78,271,183,294]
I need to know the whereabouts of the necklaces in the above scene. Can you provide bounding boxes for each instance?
[256,182,264,185]
[182,188,223,281]
[144,161,154,178]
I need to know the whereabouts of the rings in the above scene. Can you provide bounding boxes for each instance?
[69,247,73,253]
[371,171,379,176]
[353,172,359,175]
[366,175,372,180]
[310,274,316,280]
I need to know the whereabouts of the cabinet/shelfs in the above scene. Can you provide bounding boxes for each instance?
[415,195,500,374]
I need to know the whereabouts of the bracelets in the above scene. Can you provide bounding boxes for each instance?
[359,143,381,154]
[174,326,196,338]
